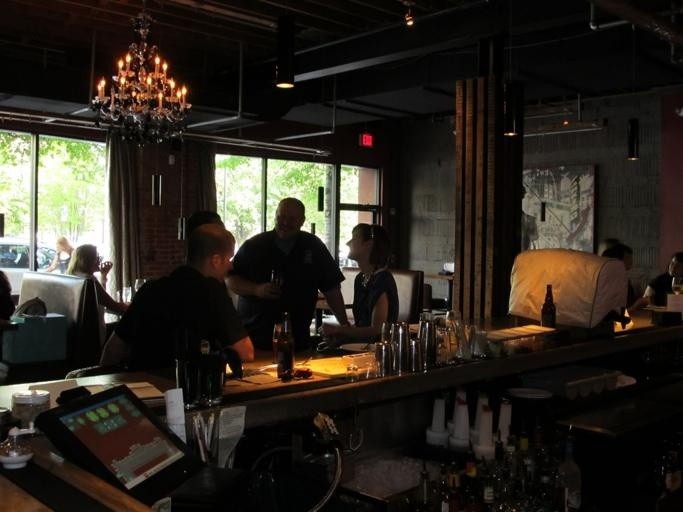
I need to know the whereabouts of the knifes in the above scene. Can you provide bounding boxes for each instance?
[172,352,225,467]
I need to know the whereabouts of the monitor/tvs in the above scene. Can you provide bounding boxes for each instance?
[34,384,205,508]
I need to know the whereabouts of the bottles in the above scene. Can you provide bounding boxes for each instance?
[540,284,556,327]
[274,311,295,381]
[418,389,564,512]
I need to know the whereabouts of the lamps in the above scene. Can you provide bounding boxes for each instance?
[88,0,193,241]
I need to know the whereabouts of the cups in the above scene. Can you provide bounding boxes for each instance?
[11,387,52,434]
[671,277,683,294]
[375,310,488,376]
[271,270,284,287]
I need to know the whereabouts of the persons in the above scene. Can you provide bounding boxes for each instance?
[601,243,650,314]
[183,209,228,295]
[96,223,254,373]
[228,197,350,352]
[42,236,73,275]
[643,252,682,301]
[64,244,130,346]
[316,223,398,343]
